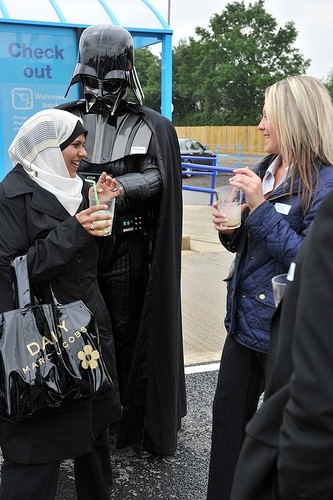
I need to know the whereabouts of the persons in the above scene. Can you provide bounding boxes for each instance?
[0,108,125,500]
[208,76,333,500]
[40,23,188,459]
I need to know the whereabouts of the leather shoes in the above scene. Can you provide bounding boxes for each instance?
[131,447,152,459]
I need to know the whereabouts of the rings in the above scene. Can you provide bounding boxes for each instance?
[245,181,251,186]
[90,224,95,230]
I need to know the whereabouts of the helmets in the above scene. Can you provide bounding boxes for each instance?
[64,24,145,116]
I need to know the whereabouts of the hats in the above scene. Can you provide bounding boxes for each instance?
[8,109,88,216]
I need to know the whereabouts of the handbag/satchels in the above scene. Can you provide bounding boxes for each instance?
[0,251,114,424]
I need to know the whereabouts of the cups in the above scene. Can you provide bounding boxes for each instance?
[271,273,289,308]
[217,199,242,229]
[89,197,115,237]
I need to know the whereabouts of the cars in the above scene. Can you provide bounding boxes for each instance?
[178,138,216,178]
